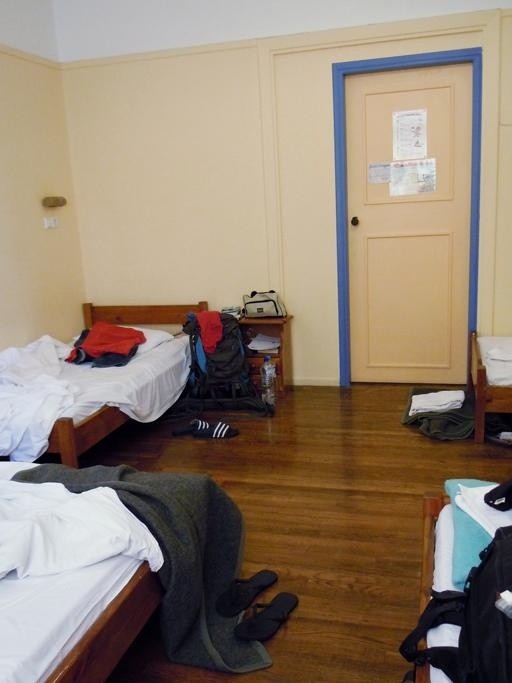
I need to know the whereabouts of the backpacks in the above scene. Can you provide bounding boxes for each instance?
[397,526,511,682]
[184,311,263,412]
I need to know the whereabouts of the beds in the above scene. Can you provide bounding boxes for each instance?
[0,460,210,682]
[470,331,512,442]
[0,300,208,467]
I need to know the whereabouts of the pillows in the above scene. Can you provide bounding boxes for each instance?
[72,323,174,358]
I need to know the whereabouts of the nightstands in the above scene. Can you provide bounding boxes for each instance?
[237,314,294,398]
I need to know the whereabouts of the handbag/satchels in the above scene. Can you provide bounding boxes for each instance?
[243,289,287,319]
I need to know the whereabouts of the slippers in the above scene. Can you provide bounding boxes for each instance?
[172,418,213,437]
[216,569,278,617]
[234,591,298,641]
[193,421,239,438]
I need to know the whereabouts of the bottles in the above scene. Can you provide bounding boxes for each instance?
[261,356,276,406]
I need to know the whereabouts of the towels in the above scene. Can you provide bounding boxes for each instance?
[408,389,466,416]
